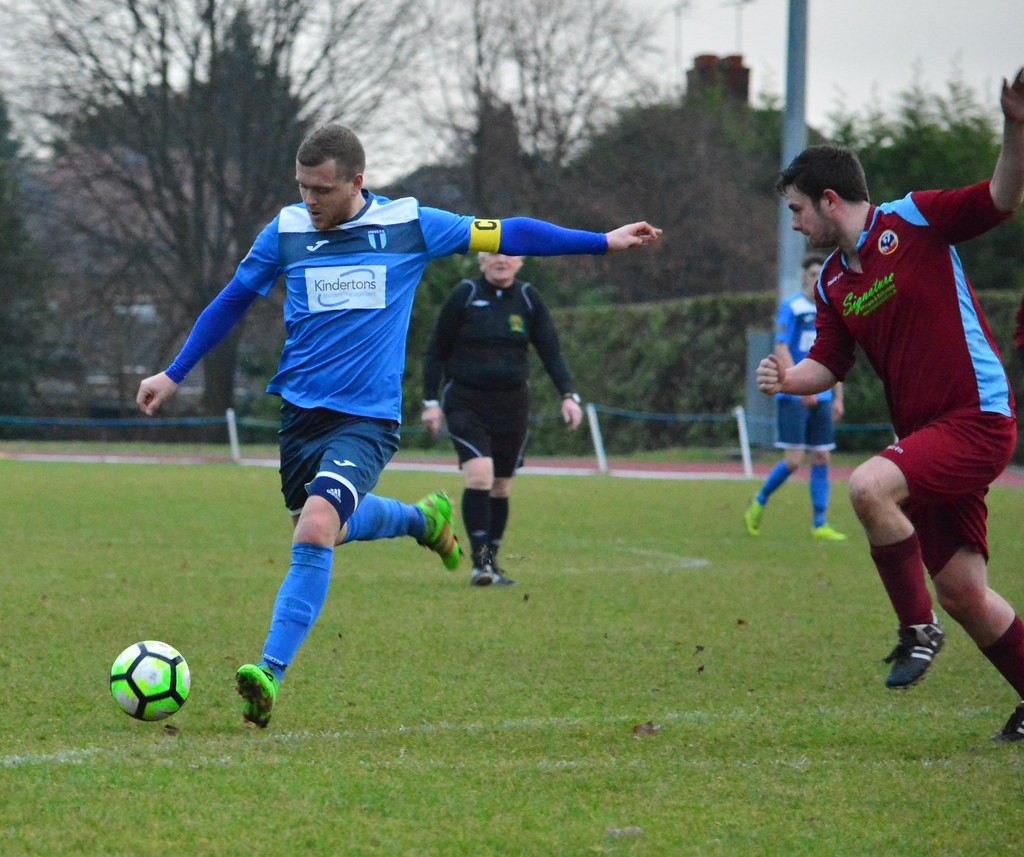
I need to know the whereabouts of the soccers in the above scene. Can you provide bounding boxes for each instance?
[109,640,191,721]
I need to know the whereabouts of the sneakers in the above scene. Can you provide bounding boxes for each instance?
[745,502,762,539]
[233,661,277,730]
[812,525,845,542]
[885,617,947,691]
[490,567,515,587]
[994,701,1023,744]
[470,562,495,585]
[413,491,459,574]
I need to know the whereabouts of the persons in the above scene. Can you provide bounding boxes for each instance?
[419,249,581,588]
[134,125,665,736]
[755,65,1024,744]
[743,252,846,540]
